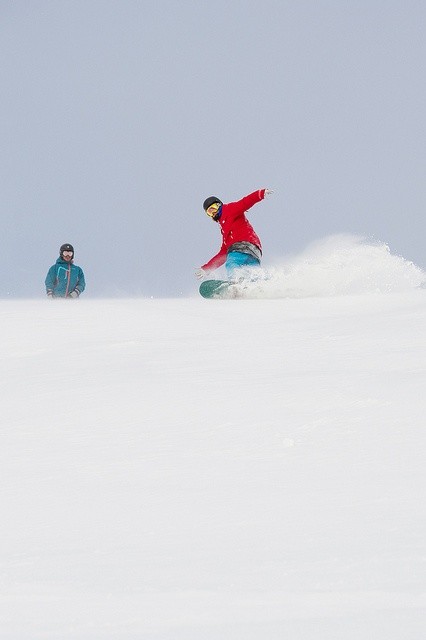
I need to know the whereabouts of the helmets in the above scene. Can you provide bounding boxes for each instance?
[59,243,74,261]
[203,196,222,221]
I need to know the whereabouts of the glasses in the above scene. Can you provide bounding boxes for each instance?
[206,202,220,217]
[61,250,73,257]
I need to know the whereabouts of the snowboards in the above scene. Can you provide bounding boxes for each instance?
[201,280,242,299]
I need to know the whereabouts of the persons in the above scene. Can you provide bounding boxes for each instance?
[193,188,274,284]
[45,242,86,300]
